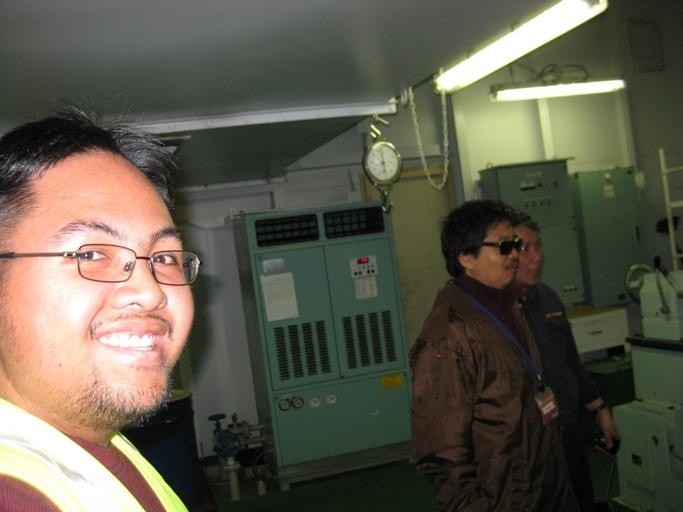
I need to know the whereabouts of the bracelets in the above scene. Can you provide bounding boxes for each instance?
[591,400,607,415]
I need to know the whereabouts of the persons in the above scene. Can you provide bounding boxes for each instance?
[514,211,621,512]
[408,200,580,512]
[0,104,202,512]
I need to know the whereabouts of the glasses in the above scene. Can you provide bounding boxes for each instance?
[0,243,201,286]
[466,239,522,254]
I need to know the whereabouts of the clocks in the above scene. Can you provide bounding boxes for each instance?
[362,141,402,185]
